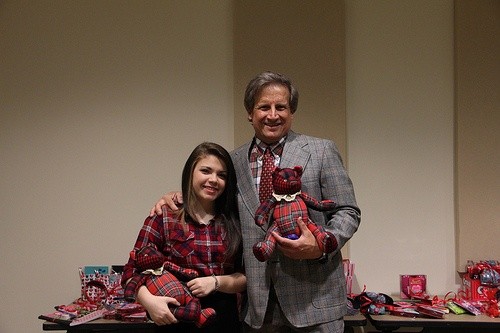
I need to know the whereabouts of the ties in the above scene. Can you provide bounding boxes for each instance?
[258,147,275,225]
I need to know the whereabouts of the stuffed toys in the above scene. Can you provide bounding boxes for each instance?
[252,166,338,262]
[124,243,216,333]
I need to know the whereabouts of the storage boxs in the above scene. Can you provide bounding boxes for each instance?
[462,275,500,303]
[402,274,426,300]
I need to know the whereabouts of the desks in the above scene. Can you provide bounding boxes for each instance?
[43,298,368,333]
[367,300,500,333]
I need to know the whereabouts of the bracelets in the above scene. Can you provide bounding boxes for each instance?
[212,275,220,290]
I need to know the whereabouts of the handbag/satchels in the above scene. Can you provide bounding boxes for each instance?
[78,267,125,305]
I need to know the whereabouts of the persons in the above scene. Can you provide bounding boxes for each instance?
[150,72,361,333]
[123,142,247,333]
